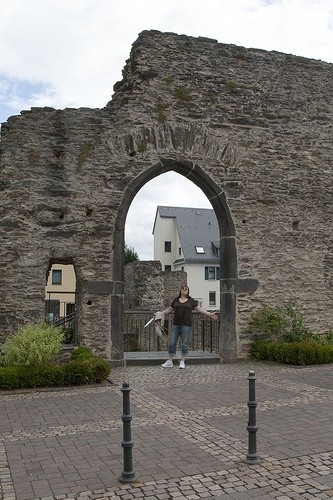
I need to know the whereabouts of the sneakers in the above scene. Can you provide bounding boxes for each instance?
[162,360,173,367]
[179,361,185,368]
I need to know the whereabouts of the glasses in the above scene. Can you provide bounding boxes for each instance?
[181,288,188,290]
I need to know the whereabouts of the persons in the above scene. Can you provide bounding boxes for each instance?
[154,285,219,369]
